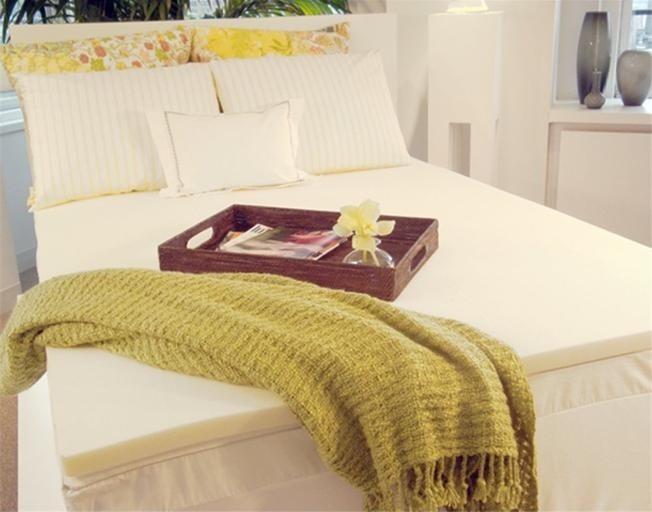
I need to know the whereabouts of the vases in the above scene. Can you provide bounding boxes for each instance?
[573,11,651,108]
[344,238,395,267]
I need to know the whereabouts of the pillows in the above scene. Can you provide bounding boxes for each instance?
[9,62,224,213]
[208,52,412,175]
[143,95,311,198]
[2,26,193,88]
[193,22,350,63]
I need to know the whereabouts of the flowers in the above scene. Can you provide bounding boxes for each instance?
[330,202,396,265]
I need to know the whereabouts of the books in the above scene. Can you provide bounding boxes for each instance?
[212,222,351,260]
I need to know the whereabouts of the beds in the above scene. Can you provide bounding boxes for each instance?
[7,14,652,510]
